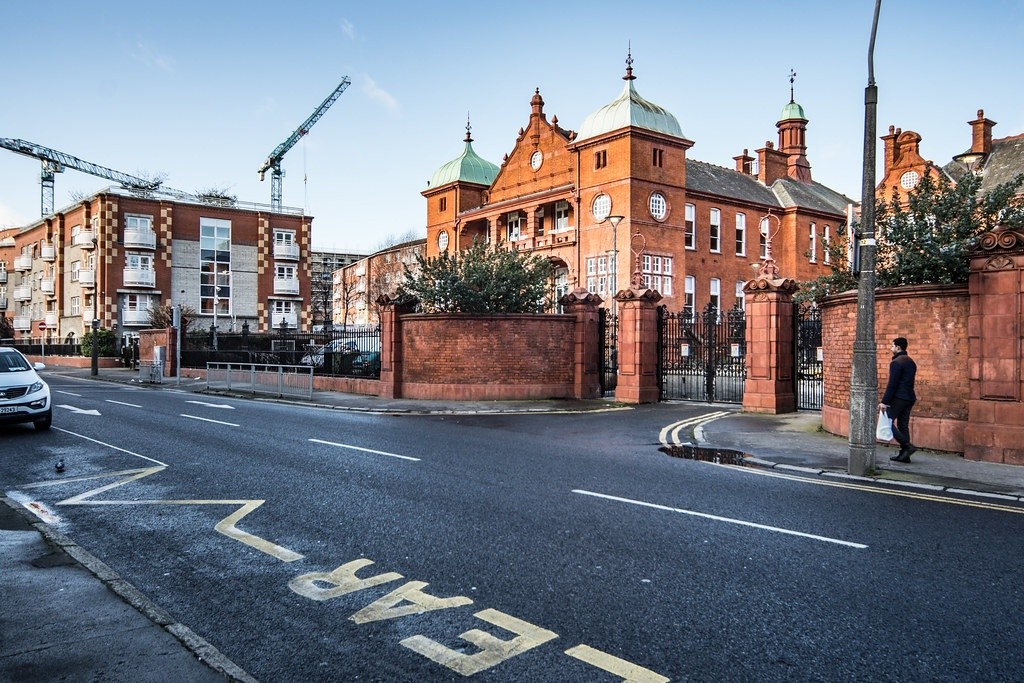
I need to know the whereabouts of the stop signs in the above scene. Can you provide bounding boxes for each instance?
[38,322,47,331]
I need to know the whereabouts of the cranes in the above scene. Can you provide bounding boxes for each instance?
[257,74,352,214]
[0,137,197,220]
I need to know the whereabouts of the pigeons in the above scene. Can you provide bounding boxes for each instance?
[55,458,66,471]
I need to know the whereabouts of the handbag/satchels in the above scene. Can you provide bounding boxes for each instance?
[876,410,893,442]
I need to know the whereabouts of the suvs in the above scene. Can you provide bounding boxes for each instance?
[0,347,54,431]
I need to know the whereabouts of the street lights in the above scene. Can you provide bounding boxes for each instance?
[91,237,100,378]
[604,212,625,366]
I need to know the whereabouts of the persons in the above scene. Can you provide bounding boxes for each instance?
[877,338,918,463]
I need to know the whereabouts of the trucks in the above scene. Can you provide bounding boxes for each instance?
[303,338,382,376]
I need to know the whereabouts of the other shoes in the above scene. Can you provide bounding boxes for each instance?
[890,450,911,463]
[897,442,916,460]
